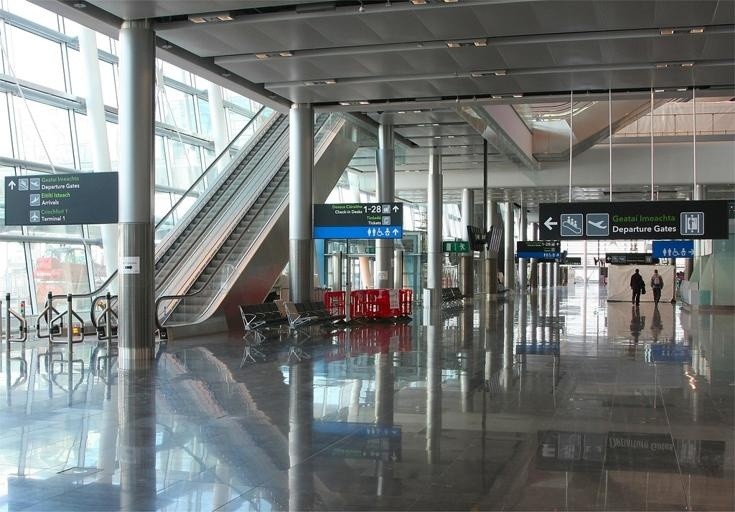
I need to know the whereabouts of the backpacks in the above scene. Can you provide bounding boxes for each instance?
[653,278,660,285]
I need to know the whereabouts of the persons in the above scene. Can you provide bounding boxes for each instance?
[631,268,645,306]
[649,306,664,342]
[651,269,664,305]
[630,307,645,344]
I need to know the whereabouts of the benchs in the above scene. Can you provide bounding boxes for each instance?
[441,287,467,305]
[239,301,346,339]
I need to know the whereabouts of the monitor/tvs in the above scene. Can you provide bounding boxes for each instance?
[467,226,483,251]
[486,224,503,253]
